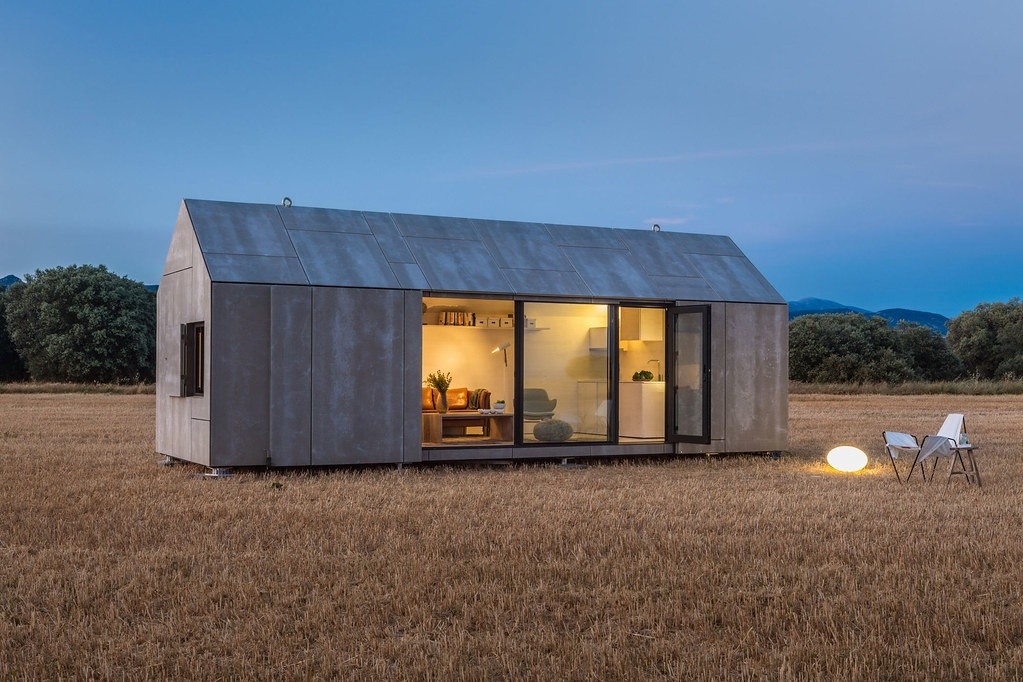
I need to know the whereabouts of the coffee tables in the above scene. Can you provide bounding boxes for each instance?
[422,412,514,444]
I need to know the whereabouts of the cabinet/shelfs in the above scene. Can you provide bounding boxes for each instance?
[621,307,664,342]
[577,379,664,438]
[589,327,628,352]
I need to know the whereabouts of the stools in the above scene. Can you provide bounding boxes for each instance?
[945,445,982,487]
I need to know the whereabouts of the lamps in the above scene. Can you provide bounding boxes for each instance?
[491,341,511,367]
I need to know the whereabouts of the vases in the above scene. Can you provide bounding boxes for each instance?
[436,390,447,414]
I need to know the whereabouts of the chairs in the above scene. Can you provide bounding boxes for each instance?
[882,414,970,484]
[514,388,557,422]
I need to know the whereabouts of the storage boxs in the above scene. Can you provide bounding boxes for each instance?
[487,317,499,327]
[528,319,537,328]
[500,318,512,327]
[475,317,487,326]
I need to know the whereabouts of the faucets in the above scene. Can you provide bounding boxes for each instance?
[647,359,665,382]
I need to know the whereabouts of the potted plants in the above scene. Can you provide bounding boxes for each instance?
[493,400,506,413]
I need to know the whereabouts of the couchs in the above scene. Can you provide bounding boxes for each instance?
[422,387,492,437]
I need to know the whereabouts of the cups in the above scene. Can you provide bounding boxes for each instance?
[478,409,503,414]
